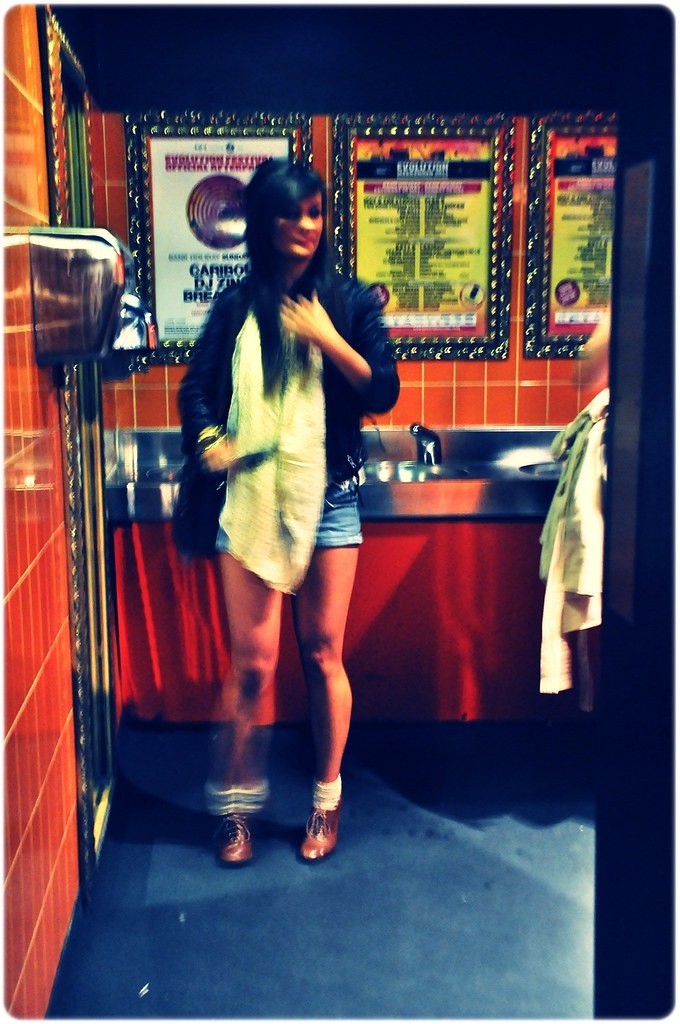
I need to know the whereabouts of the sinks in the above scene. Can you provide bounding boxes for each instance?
[517,462,564,478]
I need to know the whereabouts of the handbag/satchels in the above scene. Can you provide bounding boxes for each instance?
[171,388,227,559]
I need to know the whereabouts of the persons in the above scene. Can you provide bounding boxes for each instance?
[171,156,401,867]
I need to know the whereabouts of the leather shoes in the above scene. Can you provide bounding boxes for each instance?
[219,812,252,864]
[300,794,342,862]
